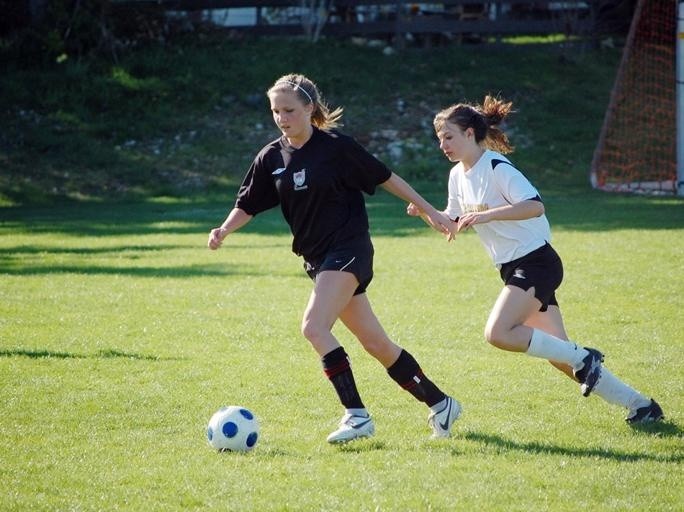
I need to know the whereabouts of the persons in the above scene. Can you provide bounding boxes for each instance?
[208,73,460,444]
[406,93,665,426]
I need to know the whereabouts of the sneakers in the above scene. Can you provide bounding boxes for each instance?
[572,346,605,396]
[427,395,462,440]
[624,398,664,425]
[327,412,376,445]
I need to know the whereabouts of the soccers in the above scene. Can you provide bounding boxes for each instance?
[208,405,259,454]
